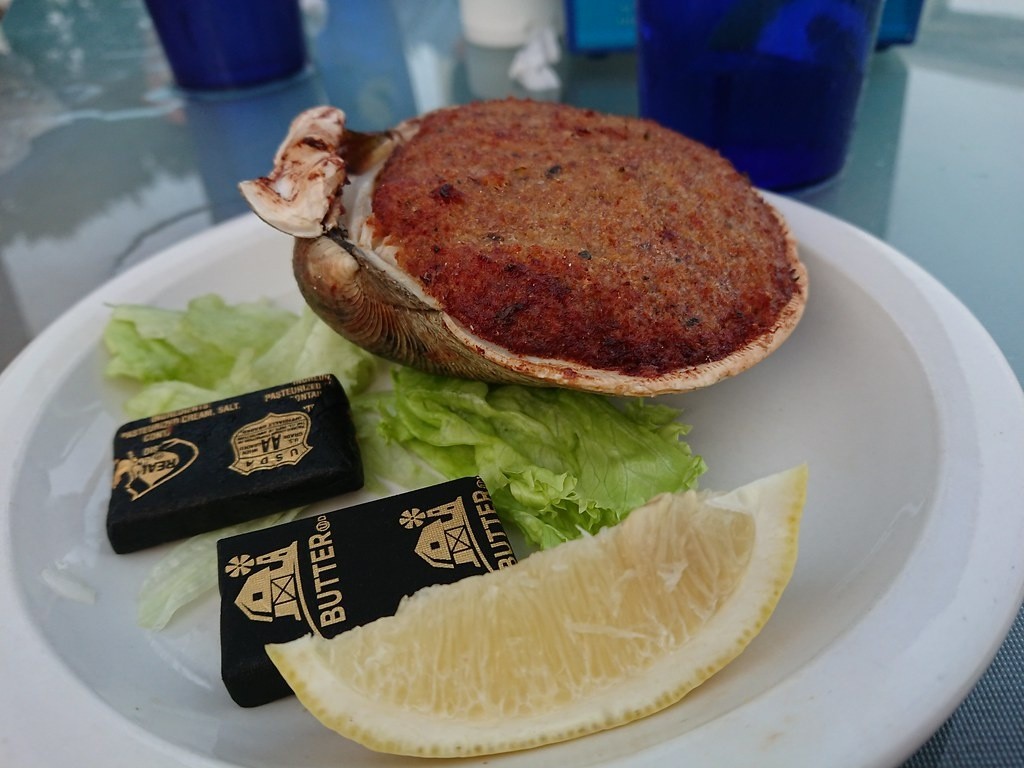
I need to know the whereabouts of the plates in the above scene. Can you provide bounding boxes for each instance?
[1,187,1024,768]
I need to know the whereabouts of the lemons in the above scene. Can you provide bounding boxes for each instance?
[262,459,812,758]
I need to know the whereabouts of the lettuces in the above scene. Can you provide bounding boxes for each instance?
[96,292,707,629]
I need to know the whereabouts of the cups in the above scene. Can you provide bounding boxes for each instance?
[636,0,886,194]
[454,2,570,49]
[142,0,312,103]
